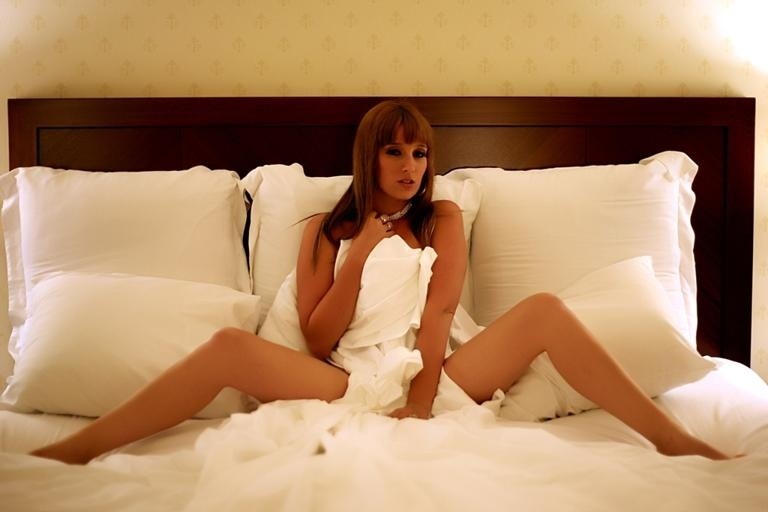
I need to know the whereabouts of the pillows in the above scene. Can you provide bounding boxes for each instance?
[0,168,253,296]
[441,152,699,349]
[506,256,717,428]
[242,162,481,331]
[2,274,263,419]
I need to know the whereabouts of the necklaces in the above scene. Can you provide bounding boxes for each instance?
[387,202,412,221]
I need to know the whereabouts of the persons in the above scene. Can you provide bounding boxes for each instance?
[29,101,746,465]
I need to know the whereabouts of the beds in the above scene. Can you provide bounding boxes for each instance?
[0,94,768,512]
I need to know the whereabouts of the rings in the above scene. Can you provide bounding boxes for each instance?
[380,216,386,222]
[386,223,391,230]
[409,414,420,418]
